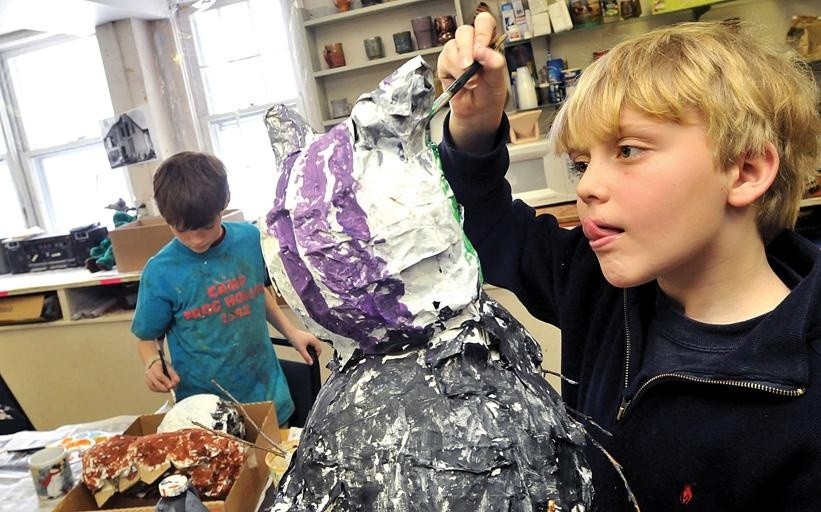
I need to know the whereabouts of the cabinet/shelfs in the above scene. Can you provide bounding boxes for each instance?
[0,203,245,431]
[292,2,821,228]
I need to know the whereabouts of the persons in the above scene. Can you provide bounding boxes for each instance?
[432,6,819,512]
[127,149,323,438]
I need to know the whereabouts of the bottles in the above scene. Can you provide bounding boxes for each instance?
[152,473,211,512]
[27,444,87,506]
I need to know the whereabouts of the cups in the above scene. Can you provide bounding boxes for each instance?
[363,36,384,62]
[322,42,347,70]
[411,15,436,50]
[393,31,414,55]
[330,97,353,119]
[516,66,539,111]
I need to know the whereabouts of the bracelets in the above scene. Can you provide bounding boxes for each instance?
[145,354,168,370]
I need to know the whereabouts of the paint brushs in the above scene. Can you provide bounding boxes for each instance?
[154,338,178,411]
[432,28,505,118]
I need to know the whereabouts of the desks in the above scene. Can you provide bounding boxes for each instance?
[0,428,303,511]
